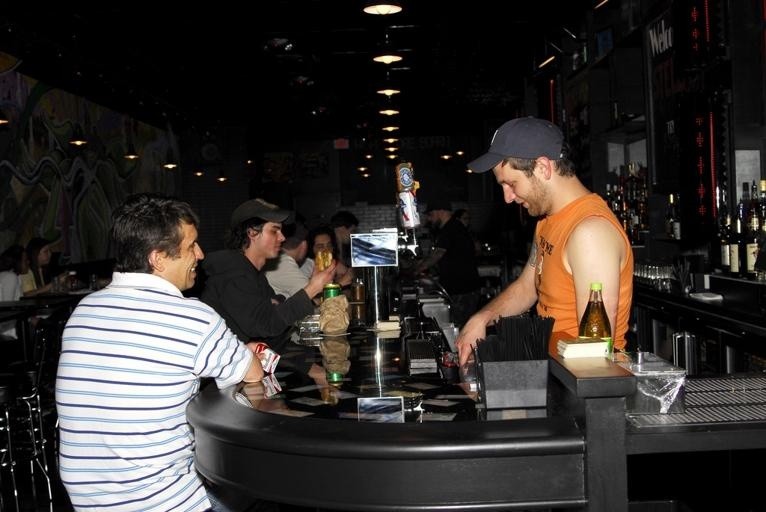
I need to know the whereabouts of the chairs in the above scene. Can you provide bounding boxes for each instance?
[0,327,54,512]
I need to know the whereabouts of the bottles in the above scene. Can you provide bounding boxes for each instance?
[577,284,613,360]
[717,179,766,280]
[662,191,682,241]
[604,168,648,244]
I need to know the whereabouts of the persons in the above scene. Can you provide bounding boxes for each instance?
[269,345,343,407]
[454,118,632,372]
[202,197,335,341]
[453,208,482,257]
[17,237,68,328]
[216,381,296,417]
[0,245,32,340]
[262,209,366,317]
[55,192,265,512]
[416,200,482,330]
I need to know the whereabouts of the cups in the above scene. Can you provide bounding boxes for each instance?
[53,272,98,294]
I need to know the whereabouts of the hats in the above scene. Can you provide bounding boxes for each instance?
[423,198,452,213]
[230,197,291,232]
[466,116,564,173]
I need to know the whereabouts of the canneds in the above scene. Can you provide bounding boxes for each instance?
[327,369,343,386]
[323,284,341,302]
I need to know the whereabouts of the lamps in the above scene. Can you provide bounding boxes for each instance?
[123,89,139,160]
[0,109,9,125]
[193,145,205,177]
[162,110,178,169]
[68,70,89,146]
[216,119,229,183]
[361,0,406,162]
